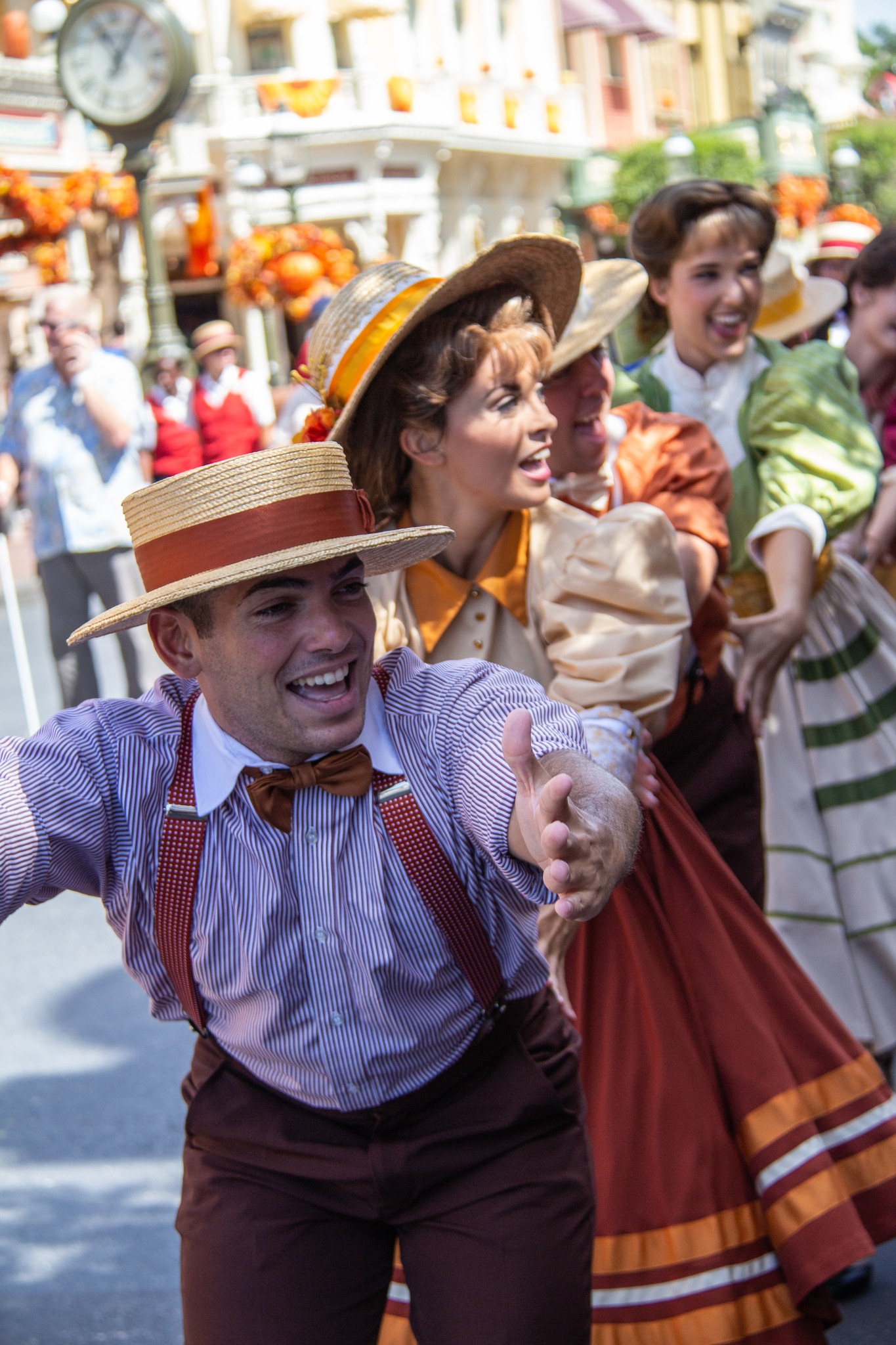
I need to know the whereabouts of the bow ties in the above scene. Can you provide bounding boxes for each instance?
[549,470,616,512]
[245,743,373,835]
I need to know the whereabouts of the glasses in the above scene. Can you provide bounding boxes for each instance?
[37,318,57,330]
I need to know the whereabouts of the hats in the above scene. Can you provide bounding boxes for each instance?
[545,257,650,380]
[803,203,882,270]
[189,320,245,360]
[749,250,849,342]
[66,440,458,648]
[288,231,583,442]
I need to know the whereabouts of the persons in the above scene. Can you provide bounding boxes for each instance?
[1,282,159,707]
[612,179,895,1097]
[533,259,768,919]
[2,442,640,1344]
[750,205,895,603]
[291,234,895,1345]
[187,321,276,464]
[135,336,206,488]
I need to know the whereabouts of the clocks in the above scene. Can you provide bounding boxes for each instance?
[56,0,199,132]
